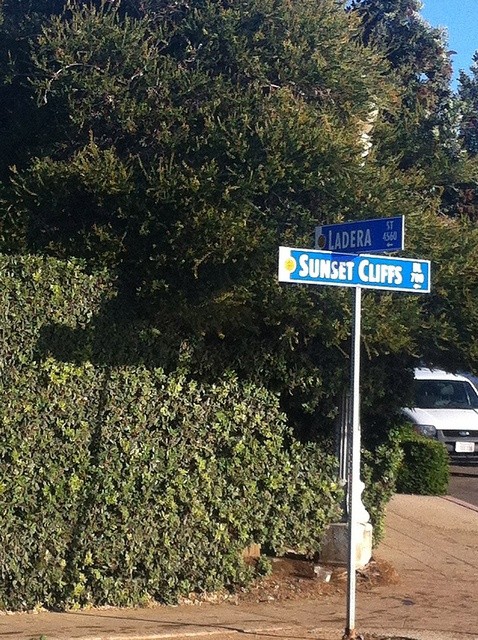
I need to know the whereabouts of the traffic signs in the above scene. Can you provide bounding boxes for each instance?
[279,246,433,295]
[313,217,404,254]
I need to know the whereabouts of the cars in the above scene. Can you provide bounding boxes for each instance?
[398,366,478,467]
